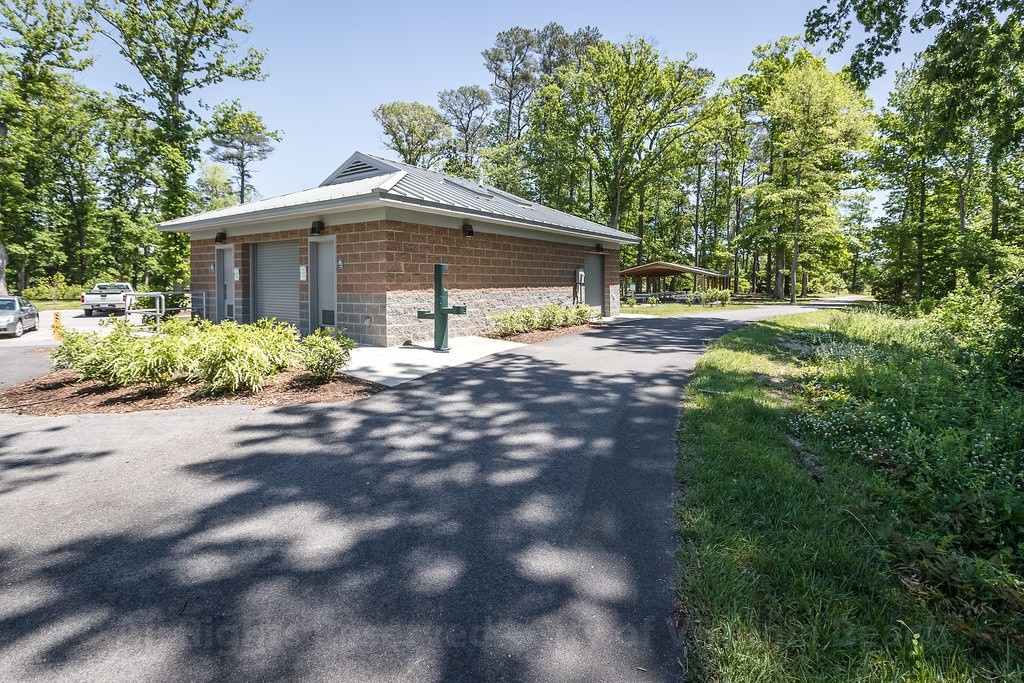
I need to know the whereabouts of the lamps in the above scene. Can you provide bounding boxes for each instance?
[311,221,324,235]
[462,225,474,238]
[596,244,603,254]
[215,232,226,244]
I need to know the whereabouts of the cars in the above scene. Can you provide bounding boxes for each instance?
[0,296,39,337]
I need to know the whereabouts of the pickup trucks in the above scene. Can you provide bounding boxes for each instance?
[80,283,135,316]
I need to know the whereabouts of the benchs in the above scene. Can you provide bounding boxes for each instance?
[633,292,688,302]
